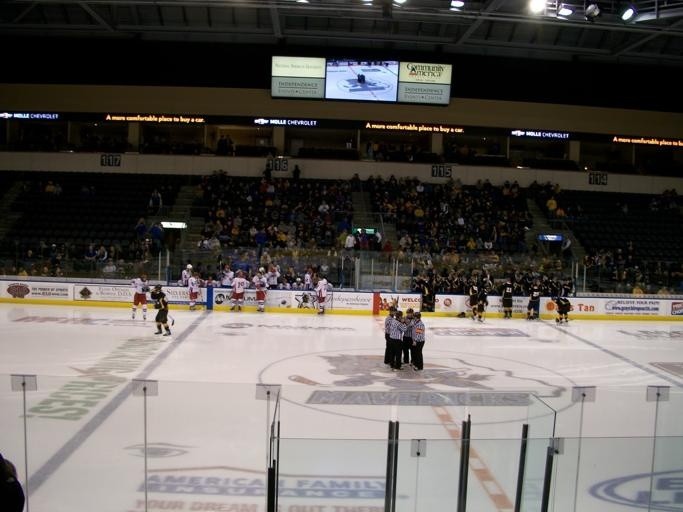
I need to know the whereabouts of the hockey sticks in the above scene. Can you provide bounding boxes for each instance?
[167,313,175,327]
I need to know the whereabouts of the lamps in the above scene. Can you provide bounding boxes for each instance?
[557,0,639,27]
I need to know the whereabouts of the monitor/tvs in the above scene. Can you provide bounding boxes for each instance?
[325,56,400,103]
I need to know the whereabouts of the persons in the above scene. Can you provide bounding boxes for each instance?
[129,275,171,337]
[390,310,405,371]
[384,305,397,364]
[0,451,27,511]
[469,282,492,321]
[187,272,199,310]
[551,293,571,325]
[525,285,541,320]
[408,311,426,371]
[229,269,268,312]
[402,308,416,366]
[314,274,327,314]
[1,130,683,297]
[502,282,514,319]
